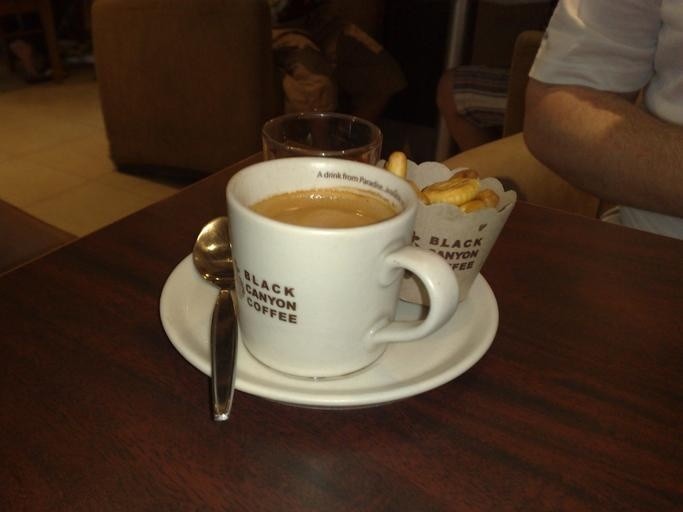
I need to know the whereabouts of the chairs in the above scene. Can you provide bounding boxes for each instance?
[0,1,69,83]
[442,134,601,218]
[501,31,544,138]
[90,1,283,177]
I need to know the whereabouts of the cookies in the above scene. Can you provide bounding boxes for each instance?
[384,149,499,212]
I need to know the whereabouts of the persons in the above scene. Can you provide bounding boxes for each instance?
[267,2,403,149]
[419,30,604,226]
[519,0,683,238]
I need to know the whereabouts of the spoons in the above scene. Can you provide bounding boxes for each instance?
[191,215,239,422]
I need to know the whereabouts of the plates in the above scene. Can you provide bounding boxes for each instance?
[160,250,500,412]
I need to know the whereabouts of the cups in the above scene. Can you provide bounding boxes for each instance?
[262,111,383,168]
[225,155,459,380]
